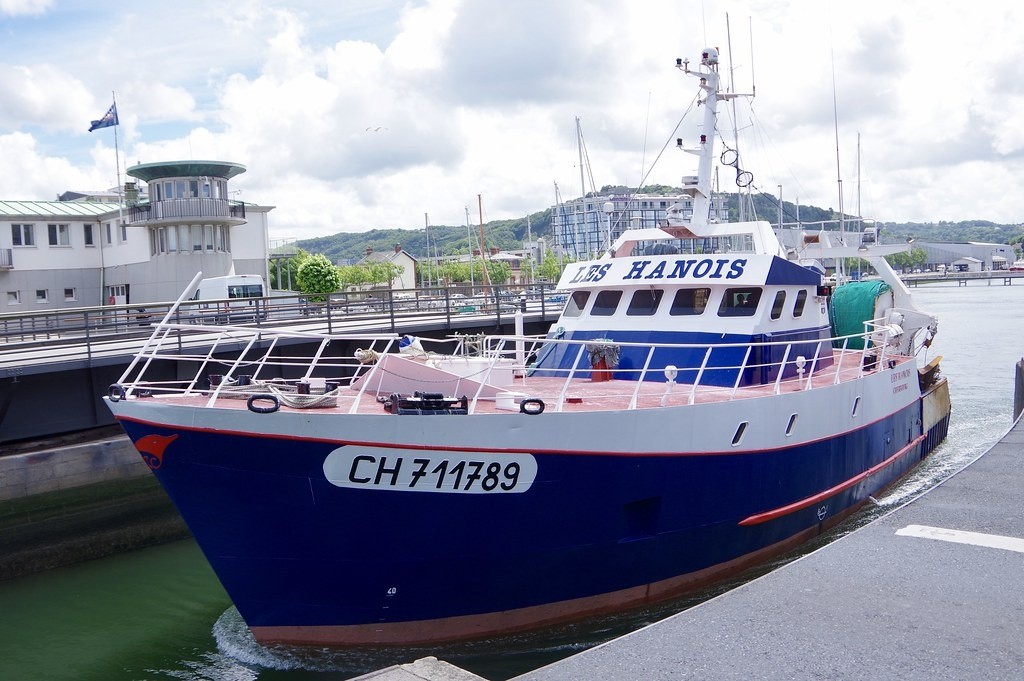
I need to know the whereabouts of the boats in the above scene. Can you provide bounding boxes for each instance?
[102,6,953,657]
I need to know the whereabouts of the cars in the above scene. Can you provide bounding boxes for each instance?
[322,287,565,314]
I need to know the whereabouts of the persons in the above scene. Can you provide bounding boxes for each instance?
[735,293,754,307]
[1001,263,1004,269]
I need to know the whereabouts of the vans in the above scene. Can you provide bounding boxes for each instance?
[188,272,269,326]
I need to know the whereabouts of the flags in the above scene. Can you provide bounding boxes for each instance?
[88,99,121,131]
